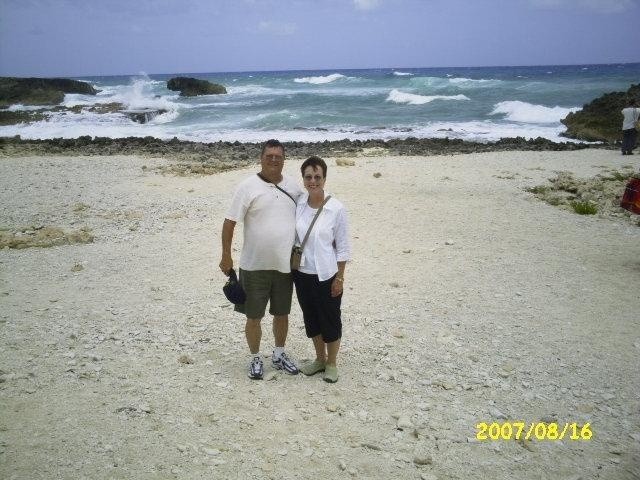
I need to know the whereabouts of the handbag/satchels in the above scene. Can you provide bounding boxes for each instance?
[288,245,303,271]
[223,266,247,306]
[633,121,640,131]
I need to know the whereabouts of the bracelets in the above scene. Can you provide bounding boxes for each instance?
[334,276,344,283]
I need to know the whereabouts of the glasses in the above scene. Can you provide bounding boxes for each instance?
[305,174,324,182]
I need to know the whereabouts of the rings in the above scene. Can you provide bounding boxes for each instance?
[225,272,228,274]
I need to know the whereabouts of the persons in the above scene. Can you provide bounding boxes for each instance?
[620,99,640,155]
[219,140,302,380]
[294,155,351,383]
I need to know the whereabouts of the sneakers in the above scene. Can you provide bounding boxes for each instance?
[271,350,301,376]
[301,358,327,375]
[622,150,635,155]
[323,361,339,384]
[246,356,265,380]
